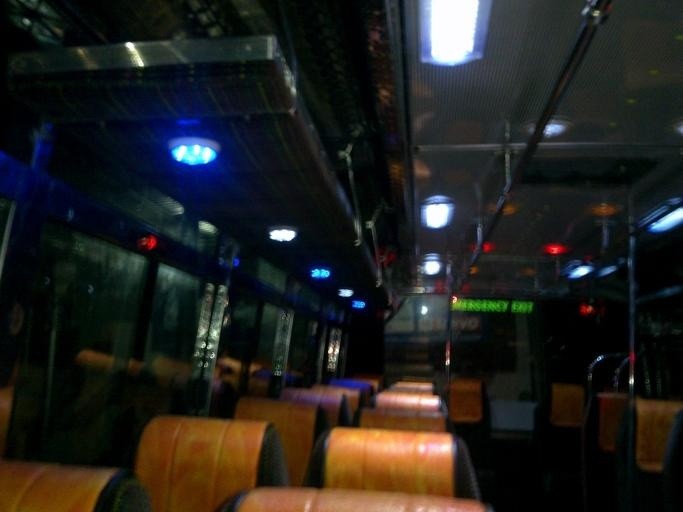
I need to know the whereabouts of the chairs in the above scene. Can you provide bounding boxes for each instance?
[547,385,682,509]
[133,416,290,510]
[229,397,320,486]
[3,458,147,511]
[216,486,486,512]
[302,426,478,499]
[280,373,451,432]
